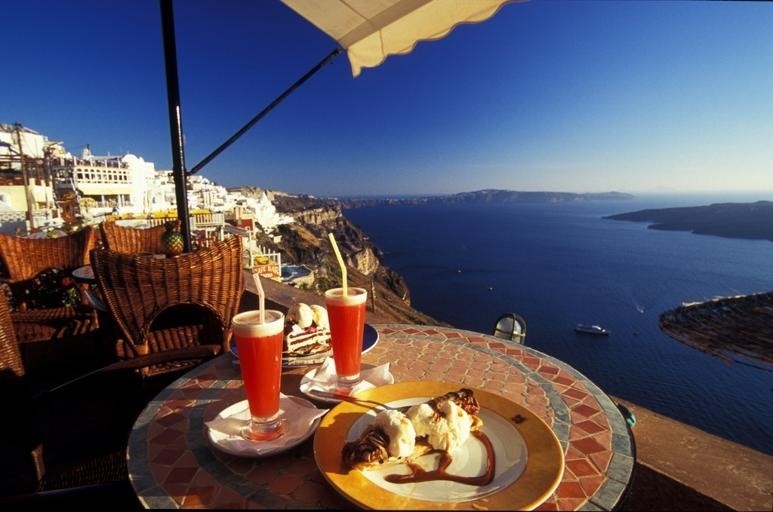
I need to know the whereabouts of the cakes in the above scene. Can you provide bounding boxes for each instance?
[341,387,482,473]
[282,303,333,363]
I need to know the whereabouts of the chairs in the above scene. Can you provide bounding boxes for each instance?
[0,222,244,512]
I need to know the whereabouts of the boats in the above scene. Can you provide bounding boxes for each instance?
[574,323,609,336]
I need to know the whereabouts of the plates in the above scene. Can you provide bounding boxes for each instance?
[312,382,564,511]
[229,316,380,370]
[298,360,396,404]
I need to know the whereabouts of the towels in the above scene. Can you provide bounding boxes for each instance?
[307,357,390,400]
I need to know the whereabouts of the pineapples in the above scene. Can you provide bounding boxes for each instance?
[160,220,184,256]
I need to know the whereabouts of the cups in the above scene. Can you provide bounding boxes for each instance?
[231,307,287,443]
[324,286,368,395]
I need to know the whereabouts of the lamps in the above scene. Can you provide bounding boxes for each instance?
[494,312,526,345]
